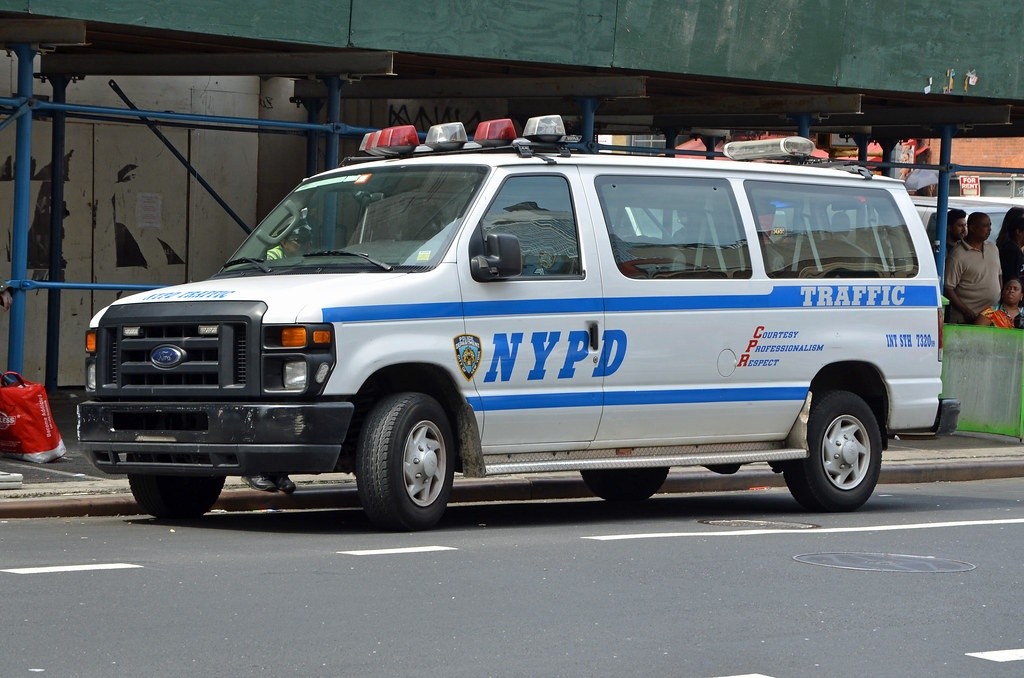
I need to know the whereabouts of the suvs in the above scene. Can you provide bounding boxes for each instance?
[74,113,962,532]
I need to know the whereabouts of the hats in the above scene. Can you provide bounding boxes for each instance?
[273,207,312,238]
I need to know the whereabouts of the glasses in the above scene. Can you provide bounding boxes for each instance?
[287,233,310,244]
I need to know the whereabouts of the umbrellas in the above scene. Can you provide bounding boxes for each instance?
[904,164,955,190]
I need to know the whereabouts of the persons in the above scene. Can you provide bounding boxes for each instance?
[913,185,936,197]
[996,207,1024,307]
[975,278,1024,328]
[239,207,314,494]
[943,212,1002,325]
[830,212,871,258]
[946,209,966,259]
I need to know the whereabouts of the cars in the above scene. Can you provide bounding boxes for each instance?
[910,195,1024,255]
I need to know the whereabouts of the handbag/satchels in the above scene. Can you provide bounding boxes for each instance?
[0,370,67,464]
